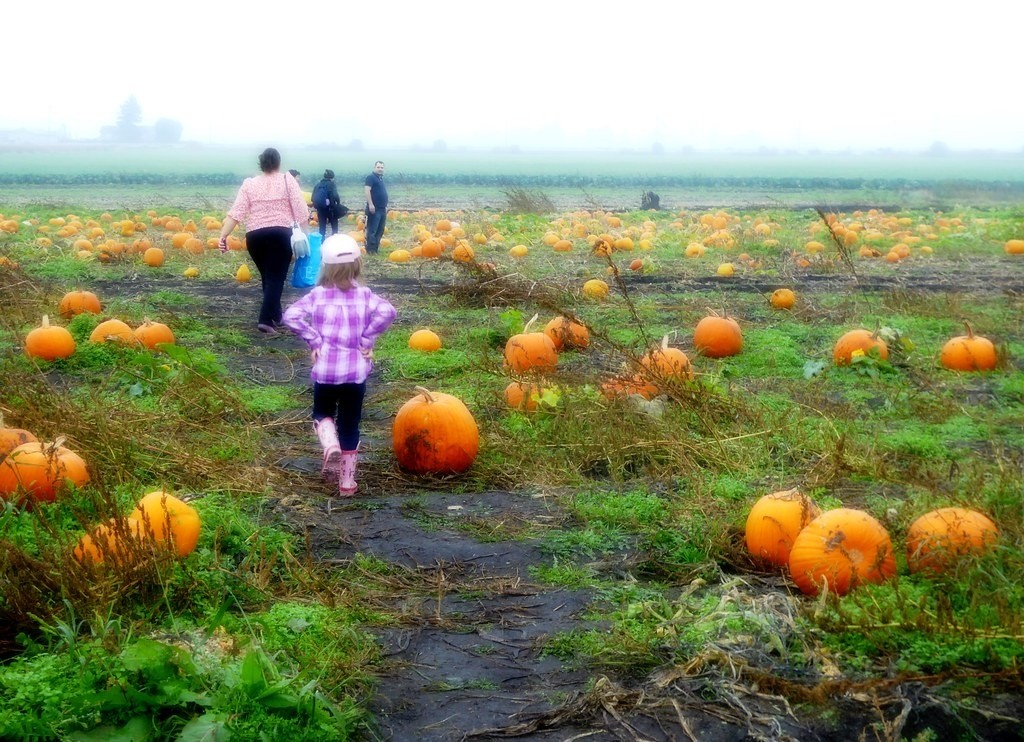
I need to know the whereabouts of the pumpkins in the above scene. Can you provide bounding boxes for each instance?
[0,210,1024,598]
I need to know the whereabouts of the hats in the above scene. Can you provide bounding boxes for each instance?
[322,233,361,264]
[324,169,334,178]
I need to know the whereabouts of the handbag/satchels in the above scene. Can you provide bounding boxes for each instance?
[291,223,322,288]
[330,201,349,220]
[290,224,310,259]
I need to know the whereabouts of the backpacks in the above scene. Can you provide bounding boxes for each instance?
[312,180,332,209]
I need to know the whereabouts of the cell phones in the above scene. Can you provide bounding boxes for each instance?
[222,238,227,254]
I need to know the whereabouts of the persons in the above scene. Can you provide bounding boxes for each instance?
[284,234,396,495]
[311,170,341,244]
[219,148,308,333]
[290,169,300,180]
[365,161,388,254]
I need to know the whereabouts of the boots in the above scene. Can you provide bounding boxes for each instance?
[339,440,360,497]
[313,418,343,480]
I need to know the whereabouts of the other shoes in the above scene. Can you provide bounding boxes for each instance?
[257,324,280,336]
